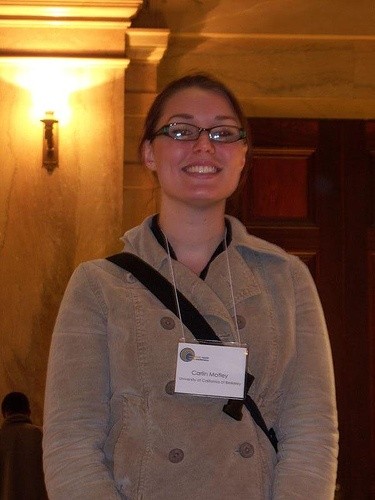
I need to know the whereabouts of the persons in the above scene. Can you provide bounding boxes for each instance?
[42,72,336,500]
[0,392,48,500]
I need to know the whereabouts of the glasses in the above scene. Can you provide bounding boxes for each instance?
[151,123,247,144]
